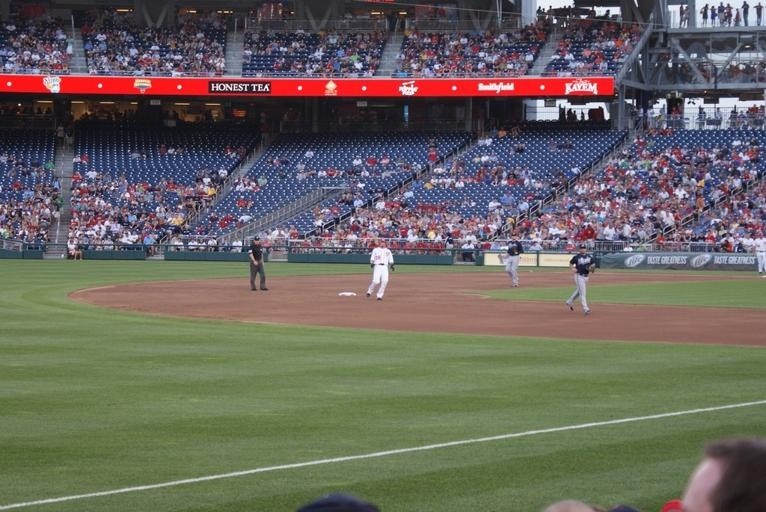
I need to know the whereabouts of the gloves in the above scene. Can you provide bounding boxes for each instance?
[390,264,395,271]
[371,263,374,267]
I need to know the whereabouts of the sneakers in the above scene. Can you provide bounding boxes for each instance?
[512,283,520,288]
[377,298,382,301]
[566,303,574,312]
[366,290,371,297]
[252,289,257,291]
[261,288,268,290]
[585,310,592,314]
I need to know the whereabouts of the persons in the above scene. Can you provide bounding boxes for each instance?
[365,241,395,299]
[169,104,612,260]
[247,236,268,290]
[565,243,596,315]
[658,53,766,84]
[678,2,763,28]
[486,101,766,254]
[1,104,271,260]
[502,233,523,287]
[1,0,646,80]
[754,232,766,274]
[683,434,766,510]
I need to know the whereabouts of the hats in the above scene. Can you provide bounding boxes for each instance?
[255,236,260,240]
[580,244,586,248]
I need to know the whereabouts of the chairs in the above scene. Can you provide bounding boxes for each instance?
[0,0,766,254]
